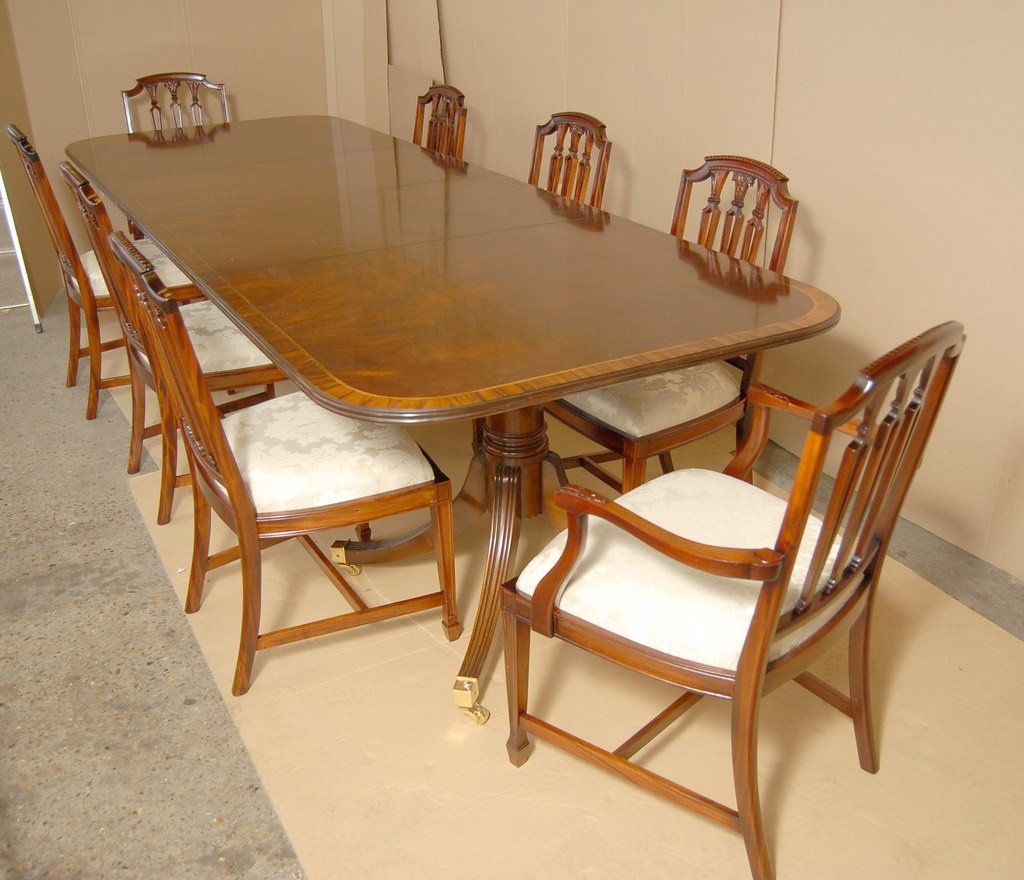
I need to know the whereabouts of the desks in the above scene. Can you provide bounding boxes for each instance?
[66,115,842,725]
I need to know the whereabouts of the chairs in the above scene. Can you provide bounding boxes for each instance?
[3,124,236,421]
[543,154,799,495]
[536,187,610,233]
[499,320,969,880]
[127,123,230,149]
[120,72,231,240]
[422,146,470,177]
[528,111,612,209]
[675,239,790,305]
[107,229,462,695]
[412,84,469,159]
[59,161,288,525]
